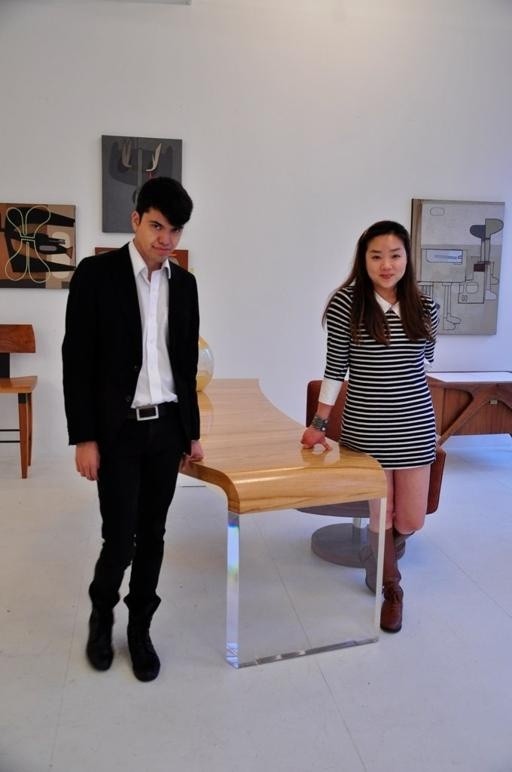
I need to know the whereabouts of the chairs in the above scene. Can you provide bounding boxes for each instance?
[295,380,446,568]
[0,324,37,479]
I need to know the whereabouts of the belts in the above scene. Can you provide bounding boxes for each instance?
[125,403,181,424]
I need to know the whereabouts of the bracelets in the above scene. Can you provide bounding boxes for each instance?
[309,415,327,432]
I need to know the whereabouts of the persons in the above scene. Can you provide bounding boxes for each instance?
[61,176,206,682]
[299,220,440,633]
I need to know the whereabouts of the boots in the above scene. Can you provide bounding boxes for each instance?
[86,581,120,670]
[365,523,404,634]
[358,523,415,595]
[124,591,163,682]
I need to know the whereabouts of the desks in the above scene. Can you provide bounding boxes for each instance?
[426,371,512,447]
[178,378,388,669]
